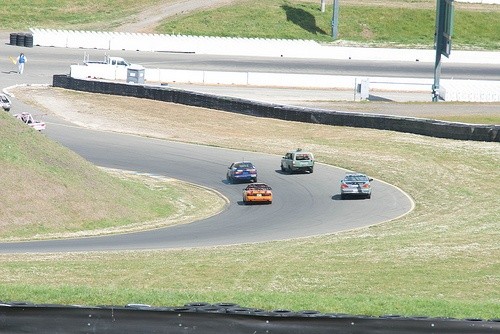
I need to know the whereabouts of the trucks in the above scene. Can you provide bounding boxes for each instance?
[82,57,127,66]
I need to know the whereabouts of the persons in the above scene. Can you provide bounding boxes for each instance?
[16,51,27,74]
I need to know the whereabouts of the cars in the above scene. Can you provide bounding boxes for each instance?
[13,112,46,132]
[226,161,258,184]
[0,94,12,112]
[241,183,273,204]
[339,174,374,200]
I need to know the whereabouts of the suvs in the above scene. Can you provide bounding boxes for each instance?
[279,148,316,175]
